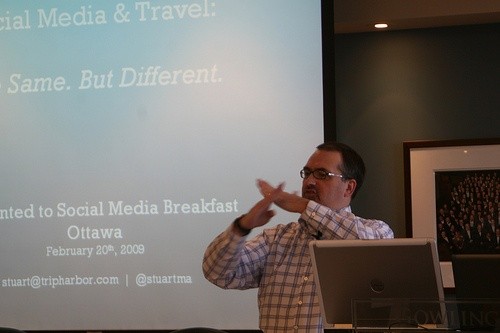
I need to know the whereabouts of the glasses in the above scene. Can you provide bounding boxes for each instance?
[300,168,345,180]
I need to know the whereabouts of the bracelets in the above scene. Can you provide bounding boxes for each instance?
[232,214,252,236]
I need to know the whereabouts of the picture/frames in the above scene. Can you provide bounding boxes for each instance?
[401,136,500,260]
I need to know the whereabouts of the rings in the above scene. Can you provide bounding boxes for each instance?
[268,192,271,196]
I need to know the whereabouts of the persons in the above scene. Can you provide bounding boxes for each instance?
[201,140,395,333]
[436,171,500,250]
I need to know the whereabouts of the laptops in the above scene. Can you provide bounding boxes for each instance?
[309,236,450,327]
